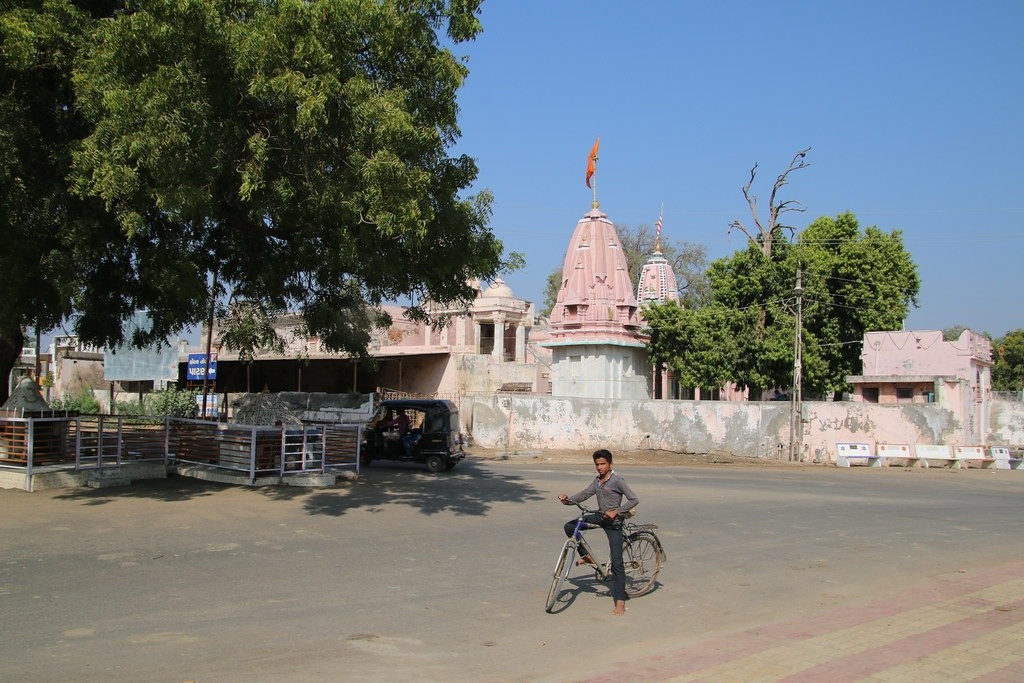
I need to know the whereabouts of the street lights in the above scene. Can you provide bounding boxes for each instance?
[789,270,804,463]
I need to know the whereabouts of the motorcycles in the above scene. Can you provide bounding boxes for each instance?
[358,399,467,474]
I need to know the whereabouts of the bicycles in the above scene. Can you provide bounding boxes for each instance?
[545,497,667,613]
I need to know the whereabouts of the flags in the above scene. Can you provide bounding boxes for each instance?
[586,138,600,191]
[655,215,663,243]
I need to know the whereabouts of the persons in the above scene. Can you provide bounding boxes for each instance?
[558,449,640,615]
[377,409,444,459]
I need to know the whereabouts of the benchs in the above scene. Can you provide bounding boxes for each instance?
[959,446,996,469]
[916,445,958,469]
[837,442,881,468]
[992,447,1024,469]
[876,443,920,468]
